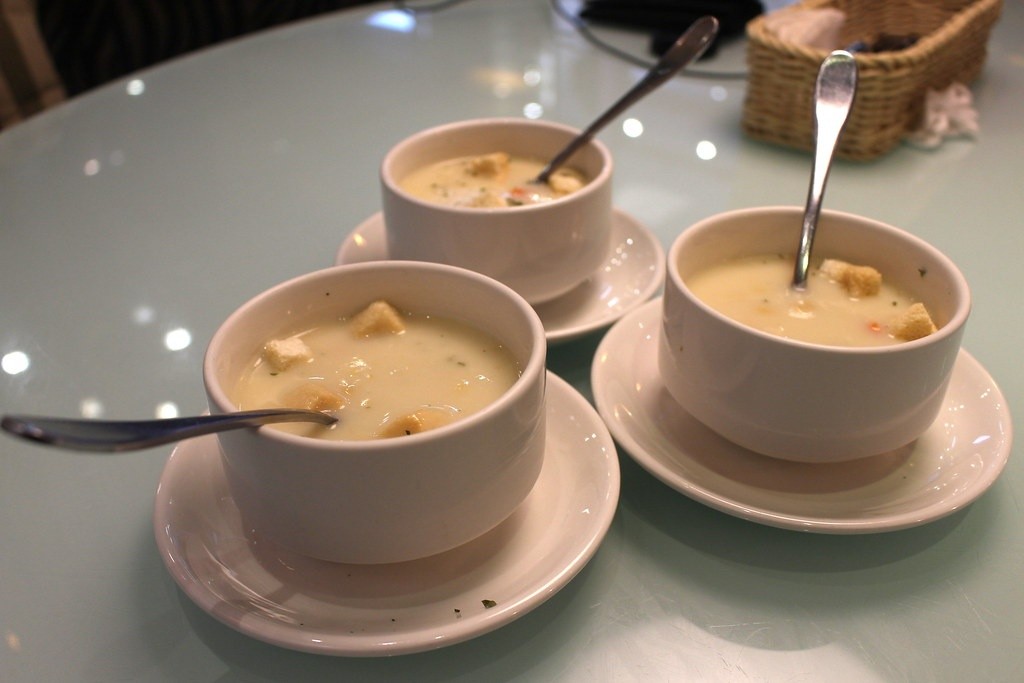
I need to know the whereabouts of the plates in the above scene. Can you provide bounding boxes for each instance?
[151,370,623,659]
[592,295,1013,536]
[336,208,664,346]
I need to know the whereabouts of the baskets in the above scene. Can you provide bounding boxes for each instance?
[741,0,1004,161]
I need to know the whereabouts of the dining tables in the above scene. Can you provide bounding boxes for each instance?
[0,0,1024,683]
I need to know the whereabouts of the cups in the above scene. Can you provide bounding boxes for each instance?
[379,116,613,305]
[657,209,970,465]
[201,260,547,566]
